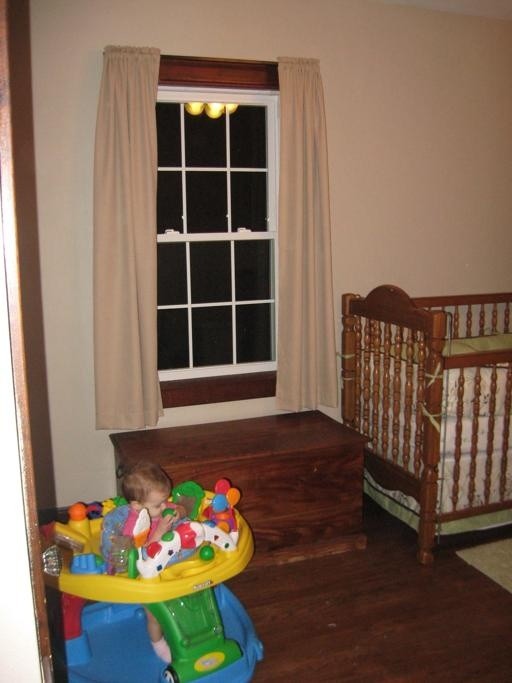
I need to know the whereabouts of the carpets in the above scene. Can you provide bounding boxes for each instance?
[455,539,512,594]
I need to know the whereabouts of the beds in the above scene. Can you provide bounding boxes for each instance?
[339,287,512,566]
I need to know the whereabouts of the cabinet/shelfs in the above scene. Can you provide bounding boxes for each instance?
[108,416,375,576]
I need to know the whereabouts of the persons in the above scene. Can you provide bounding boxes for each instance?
[107,462,188,664]
